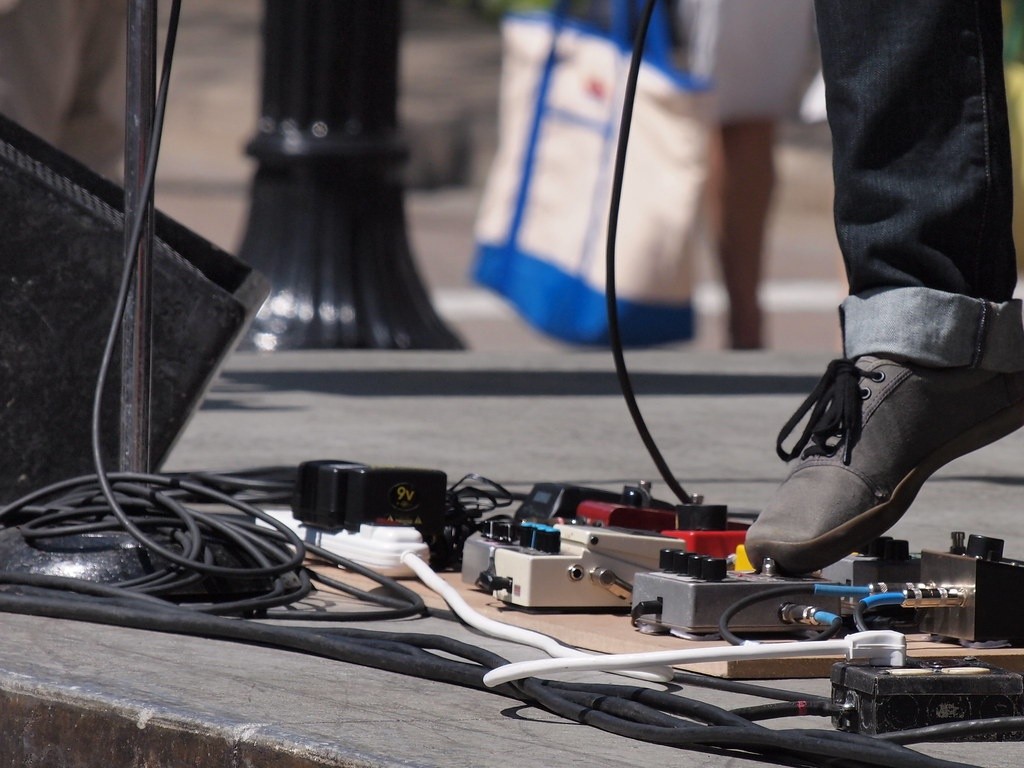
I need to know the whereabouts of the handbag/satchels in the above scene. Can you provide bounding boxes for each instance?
[470,0,716,350]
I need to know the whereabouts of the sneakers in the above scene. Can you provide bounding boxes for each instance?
[746,353,1024,573]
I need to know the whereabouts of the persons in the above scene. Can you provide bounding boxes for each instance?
[674,1,824,353]
[740,1,1024,578]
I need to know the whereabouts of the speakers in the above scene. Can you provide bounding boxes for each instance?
[0,118,271,530]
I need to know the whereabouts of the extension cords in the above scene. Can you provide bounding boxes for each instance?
[255,510,431,579]
